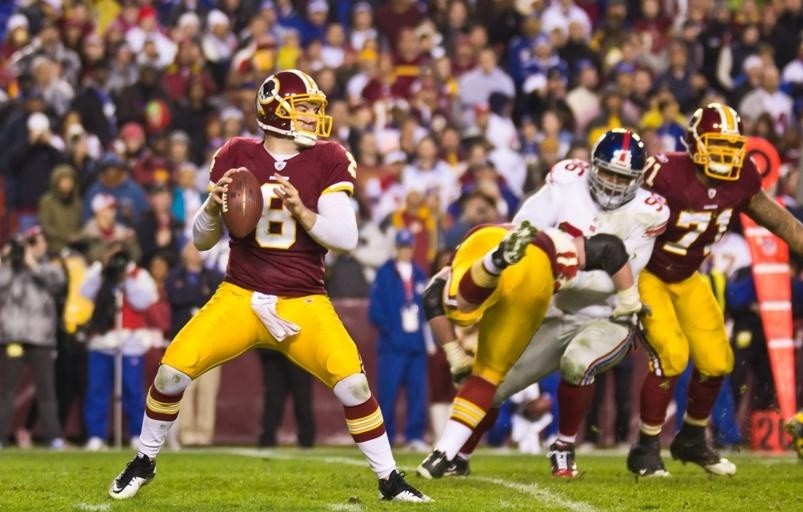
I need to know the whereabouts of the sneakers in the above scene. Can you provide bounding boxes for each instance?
[670,422,741,477]
[378,471,435,502]
[627,430,672,478]
[545,432,582,476]
[107,455,158,499]
[419,449,470,482]
[12,429,182,454]
[492,221,538,268]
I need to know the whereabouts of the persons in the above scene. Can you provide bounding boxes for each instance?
[105,70,431,502]
[624,101,803,479]
[501,126,668,479]
[0,0,803,456]
[415,219,649,482]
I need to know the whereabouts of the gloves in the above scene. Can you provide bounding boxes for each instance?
[443,338,475,391]
[610,285,653,342]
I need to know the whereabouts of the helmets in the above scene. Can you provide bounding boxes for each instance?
[587,129,646,207]
[679,102,749,183]
[254,69,332,149]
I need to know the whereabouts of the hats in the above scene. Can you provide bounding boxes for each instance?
[397,229,413,243]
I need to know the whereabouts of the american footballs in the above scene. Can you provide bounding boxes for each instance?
[225,166,262,240]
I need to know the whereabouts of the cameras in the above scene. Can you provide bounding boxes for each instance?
[108,248,129,276]
[8,241,24,269]
